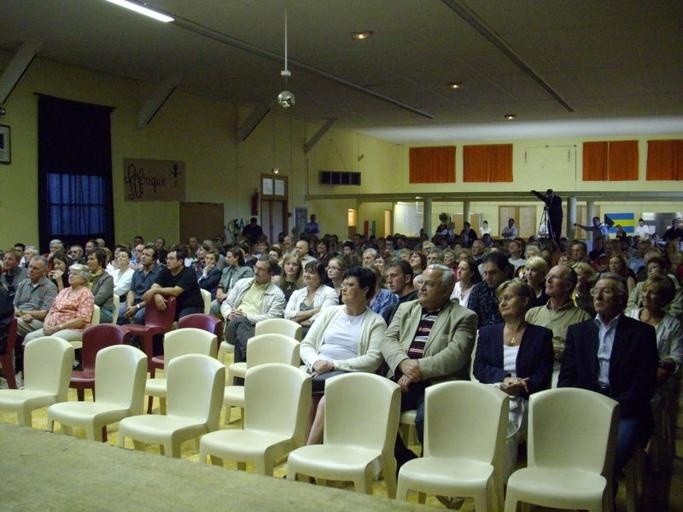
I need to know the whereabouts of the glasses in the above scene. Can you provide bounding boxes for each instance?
[590,288,612,296]
[341,282,357,287]
[328,266,341,271]
[544,274,559,280]
[165,257,175,260]
[67,271,79,276]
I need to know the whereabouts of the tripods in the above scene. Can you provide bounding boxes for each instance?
[535,207,554,241]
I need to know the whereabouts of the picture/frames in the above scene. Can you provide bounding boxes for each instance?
[0,124,12,165]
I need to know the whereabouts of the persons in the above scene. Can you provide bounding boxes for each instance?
[1,212,683,512]
[530,189,564,242]
